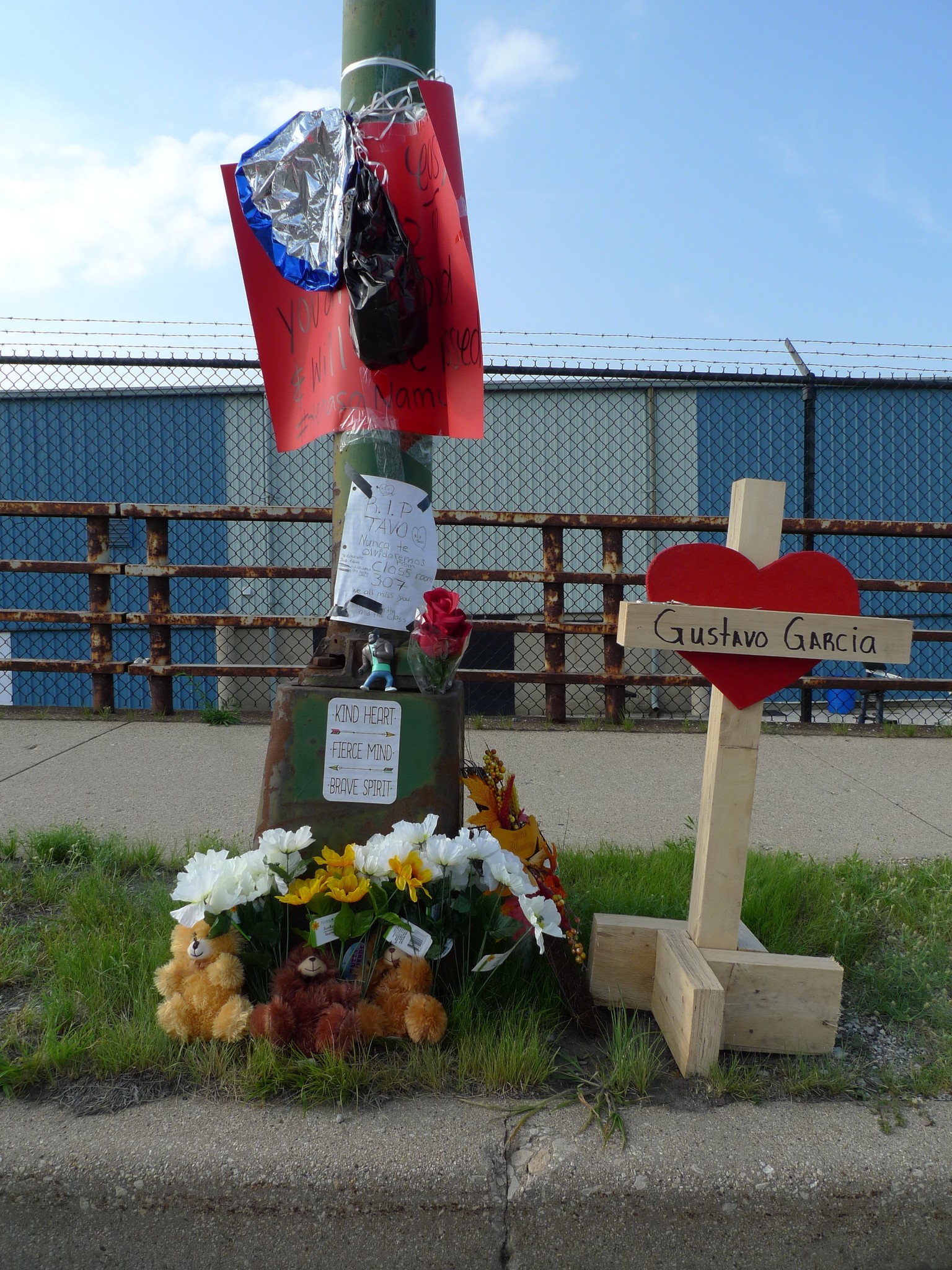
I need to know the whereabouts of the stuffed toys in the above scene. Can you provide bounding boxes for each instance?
[246,942,362,1058]
[152,906,254,1041]
[351,931,447,1044]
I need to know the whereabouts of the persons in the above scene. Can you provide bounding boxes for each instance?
[357,627,397,692]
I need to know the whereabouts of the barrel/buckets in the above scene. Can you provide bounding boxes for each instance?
[829,674,857,715]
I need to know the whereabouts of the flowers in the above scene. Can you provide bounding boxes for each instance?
[410,587,472,691]
